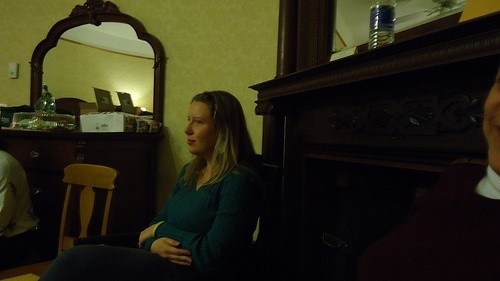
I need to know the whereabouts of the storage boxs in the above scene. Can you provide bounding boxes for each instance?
[79,113,139,132]
[0,105,36,128]
[11,112,78,130]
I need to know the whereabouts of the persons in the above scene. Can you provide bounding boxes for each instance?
[0,149,41,270]
[353,64,500,281]
[36,90,257,281]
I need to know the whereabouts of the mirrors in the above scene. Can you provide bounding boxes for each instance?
[29,0,166,119]
[276,0,500,77]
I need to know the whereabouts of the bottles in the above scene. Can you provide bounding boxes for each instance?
[368,0,396,50]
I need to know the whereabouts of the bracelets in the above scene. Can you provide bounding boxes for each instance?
[151,220,166,238]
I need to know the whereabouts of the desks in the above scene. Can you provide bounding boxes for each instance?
[0,130,166,239]
[246,12,500,281]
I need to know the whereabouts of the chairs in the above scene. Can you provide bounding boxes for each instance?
[58,163,119,257]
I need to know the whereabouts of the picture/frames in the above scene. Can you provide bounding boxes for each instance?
[116,91,136,115]
[93,87,115,113]
